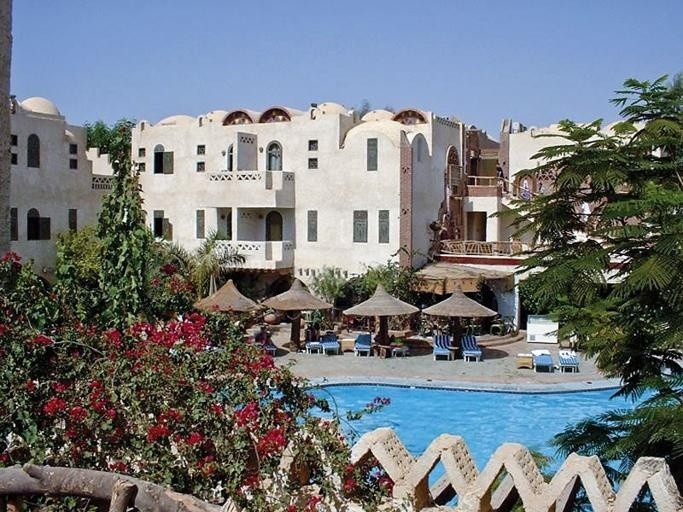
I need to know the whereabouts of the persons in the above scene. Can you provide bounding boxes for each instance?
[313,320,321,341]
[496,163,505,193]
[254,326,277,351]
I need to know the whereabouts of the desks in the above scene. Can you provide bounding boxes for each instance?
[516,352,533,369]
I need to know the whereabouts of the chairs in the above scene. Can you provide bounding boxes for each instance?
[248,329,485,363]
[532,348,580,373]
